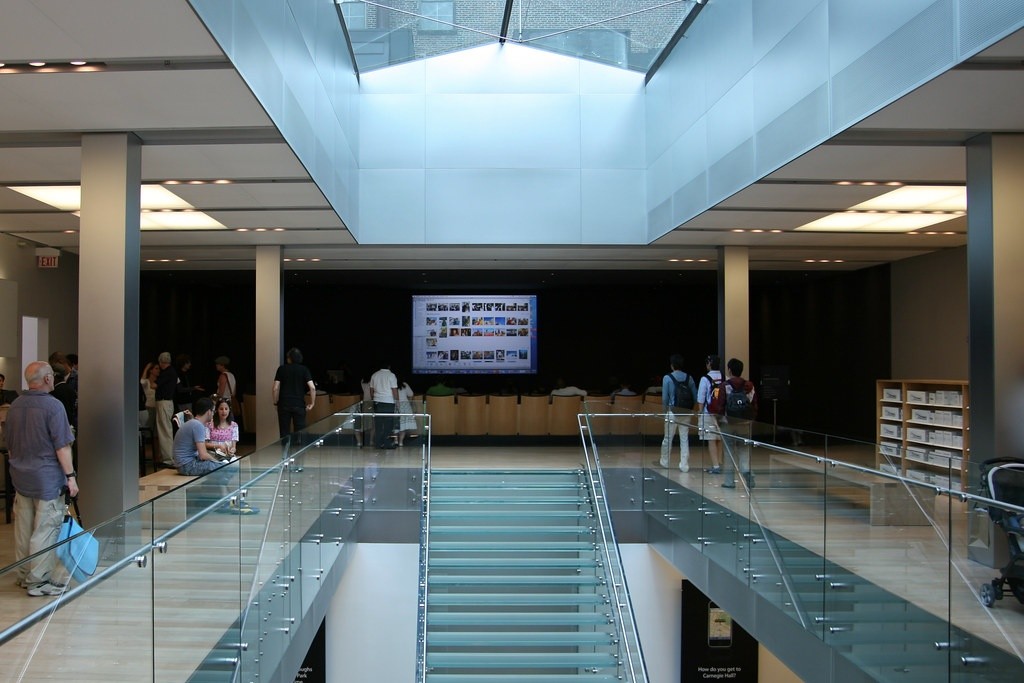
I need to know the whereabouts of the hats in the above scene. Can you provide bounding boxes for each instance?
[216,357,230,364]
[158,353,171,364]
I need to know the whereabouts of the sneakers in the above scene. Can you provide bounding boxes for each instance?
[28,579,70,596]
[704,467,722,473]
[17,579,28,589]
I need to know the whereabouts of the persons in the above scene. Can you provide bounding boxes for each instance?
[0,373,19,453]
[717,358,756,488]
[549,379,588,397]
[205,398,252,507]
[273,348,316,473]
[4,362,79,597]
[174,353,205,410]
[609,379,638,396]
[459,380,482,395]
[696,353,724,474]
[368,358,400,450]
[494,377,524,395]
[150,351,178,468]
[424,376,456,395]
[394,372,418,447]
[139,362,160,460]
[48,351,78,474]
[651,355,698,472]
[172,397,260,514]
[361,364,378,447]
[213,356,236,421]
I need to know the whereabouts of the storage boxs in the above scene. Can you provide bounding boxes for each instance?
[879,389,963,491]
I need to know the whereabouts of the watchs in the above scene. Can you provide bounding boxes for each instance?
[65,471,77,478]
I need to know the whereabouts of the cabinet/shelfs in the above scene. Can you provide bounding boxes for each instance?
[876,379,968,495]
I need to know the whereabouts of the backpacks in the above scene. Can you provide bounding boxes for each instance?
[703,375,726,414]
[727,380,749,417]
[668,373,694,413]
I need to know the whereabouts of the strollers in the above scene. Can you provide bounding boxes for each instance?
[977,456,1024,612]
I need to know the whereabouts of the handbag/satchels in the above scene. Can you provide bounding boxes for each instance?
[56,515,99,583]
[232,397,241,416]
[394,409,400,429]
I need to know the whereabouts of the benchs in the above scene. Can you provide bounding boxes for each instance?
[769,453,936,527]
[242,395,704,434]
[139,456,252,530]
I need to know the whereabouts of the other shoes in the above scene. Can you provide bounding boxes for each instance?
[747,477,755,488]
[721,484,735,488]
